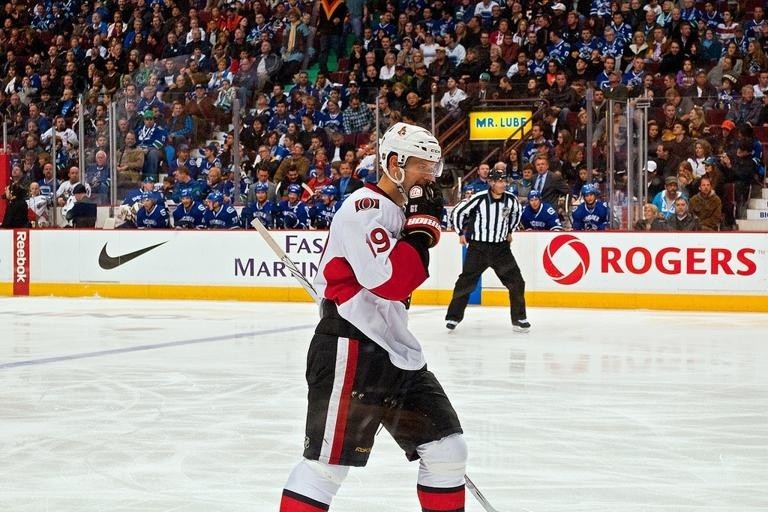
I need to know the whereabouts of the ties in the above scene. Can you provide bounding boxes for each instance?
[537,175,542,193]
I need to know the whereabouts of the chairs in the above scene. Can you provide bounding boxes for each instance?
[0,0,767,230]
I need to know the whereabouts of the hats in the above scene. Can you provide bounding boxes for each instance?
[528,189,542,199]
[720,119,736,130]
[223,164,242,174]
[581,184,598,197]
[643,160,657,174]
[143,111,154,119]
[664,176,677,185]
[702,156,717,164]
[479,73,491,81]
[551,3,567,12]
[315,159,326,169]
[195,83,204,90]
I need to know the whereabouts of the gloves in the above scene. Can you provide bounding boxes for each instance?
[405,180,444,247]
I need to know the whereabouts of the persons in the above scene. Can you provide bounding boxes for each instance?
[0,0,768,230]
[281,121,468,511]
[445,168,531,333]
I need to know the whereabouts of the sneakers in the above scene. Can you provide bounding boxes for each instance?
[512,319,531,329]
[446,319,459,330]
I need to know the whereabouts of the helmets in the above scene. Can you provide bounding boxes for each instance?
[144,176,157,183]
[487,167,507,186]
[177,144,190,154]
[379,122,444,177]
[73,184,86,194]
[202,144,217,152]
[141,184,338,206]
